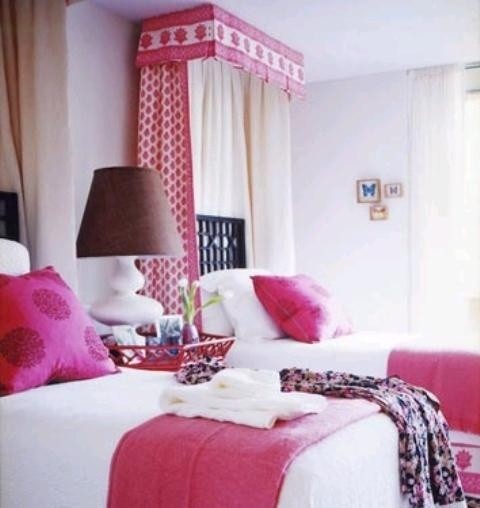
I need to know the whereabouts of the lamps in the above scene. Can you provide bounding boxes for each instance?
[74,165,187,344]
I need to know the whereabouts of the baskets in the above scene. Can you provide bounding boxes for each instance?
[102,333,236,372]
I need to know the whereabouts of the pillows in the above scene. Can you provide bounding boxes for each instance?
[201,267,286,345]
[250,269,358,342]
[3,265,120,396]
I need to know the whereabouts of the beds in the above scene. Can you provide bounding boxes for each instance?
[1,236,452,508]
[196,260,480,501]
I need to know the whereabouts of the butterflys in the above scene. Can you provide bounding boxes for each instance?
[362,183,376,197]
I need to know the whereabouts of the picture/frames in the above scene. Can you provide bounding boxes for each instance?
[355,176,405,203]
